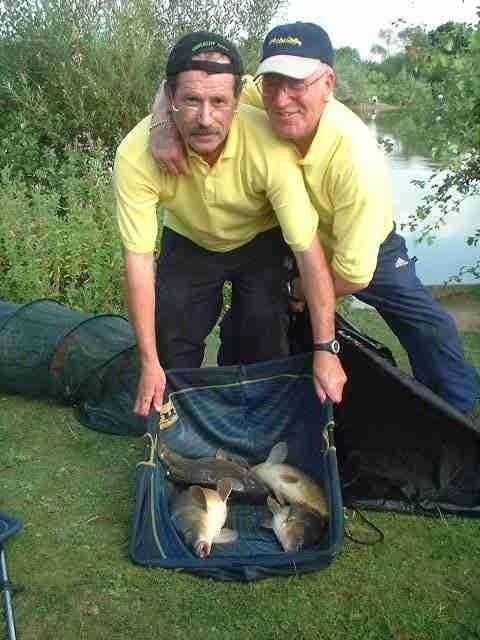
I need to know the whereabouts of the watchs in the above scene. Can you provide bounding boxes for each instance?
[311,339,340,355]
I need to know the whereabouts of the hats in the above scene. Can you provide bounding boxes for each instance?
[167,30,245,76]
[253,21,335,80]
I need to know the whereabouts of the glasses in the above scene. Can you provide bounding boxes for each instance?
[260,70,329,98]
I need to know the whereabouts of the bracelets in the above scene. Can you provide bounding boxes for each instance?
[150,119,174,131]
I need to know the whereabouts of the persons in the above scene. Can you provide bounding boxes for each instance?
[112,30,348,418]
[149,22,479,415]
